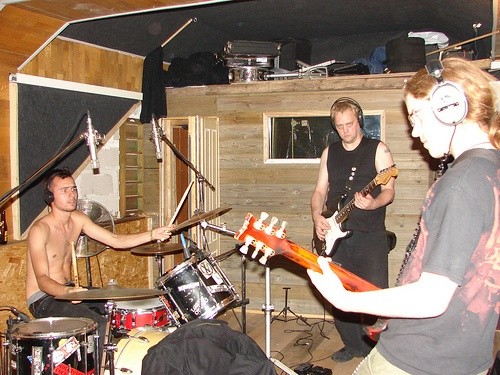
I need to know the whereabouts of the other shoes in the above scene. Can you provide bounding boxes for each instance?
[332,345,365,361]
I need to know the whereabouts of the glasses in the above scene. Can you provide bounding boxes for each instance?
[407,108,432,127]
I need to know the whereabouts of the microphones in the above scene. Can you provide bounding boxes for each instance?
[180,232,191,260]
[87,114,100,174]
[10,306,30,323]
[152,113,162,163]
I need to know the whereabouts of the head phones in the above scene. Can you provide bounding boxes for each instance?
[424,59,468,126]
[330,97,364,129]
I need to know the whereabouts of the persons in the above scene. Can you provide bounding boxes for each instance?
[26,169,176,375]
[311,97,395,362]
[349,57,500,375]
[285,117,318,159]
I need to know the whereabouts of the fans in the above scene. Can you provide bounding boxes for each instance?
[73,198,114,290]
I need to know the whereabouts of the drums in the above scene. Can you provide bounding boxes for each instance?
[101,325,175,375]
[153,251,239,319]
[6,317,101,375]
[106,299,170,332]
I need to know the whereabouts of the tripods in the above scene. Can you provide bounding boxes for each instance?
[271,287,312,327]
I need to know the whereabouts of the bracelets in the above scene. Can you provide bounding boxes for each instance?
[150,229,152,242]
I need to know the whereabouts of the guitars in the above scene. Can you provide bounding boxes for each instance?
[311,163,400,257]
[233,213,378,291]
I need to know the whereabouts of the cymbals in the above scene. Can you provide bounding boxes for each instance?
[131,243,184,255]
[170,205,233,230]
[54,284,167,300]
